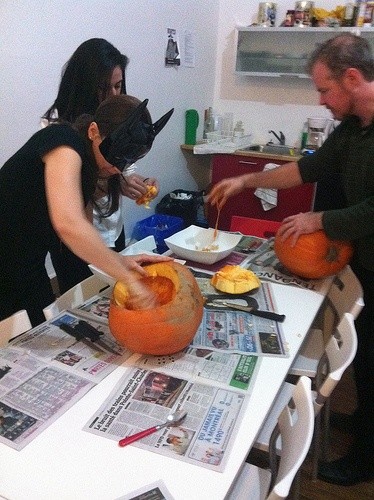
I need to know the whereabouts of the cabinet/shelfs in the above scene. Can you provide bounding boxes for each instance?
[232,25,374,80]
[209,154,318,230]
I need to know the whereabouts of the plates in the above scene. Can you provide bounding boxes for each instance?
[87,244,186,287]
[164,224,244,265]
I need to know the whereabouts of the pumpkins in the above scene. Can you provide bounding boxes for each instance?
[274,223,353,278]
[109,261,203,357]
[211,263,260,294]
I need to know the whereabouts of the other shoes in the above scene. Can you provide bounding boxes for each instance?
[317,455,374,485]
[328,411,355,434]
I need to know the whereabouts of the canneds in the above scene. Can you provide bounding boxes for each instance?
[257,2,277,28]
[292,0,315,28]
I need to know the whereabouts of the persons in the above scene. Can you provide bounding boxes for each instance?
[0,95,175,329]
[35,39,159,296]
[203,31,374,486]
[96,304,110,315]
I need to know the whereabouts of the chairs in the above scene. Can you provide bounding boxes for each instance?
[0,235,158,346]
[228,266,364,500]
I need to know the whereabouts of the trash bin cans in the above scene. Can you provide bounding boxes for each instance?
[132,214,185,255]
[161,189,203,225]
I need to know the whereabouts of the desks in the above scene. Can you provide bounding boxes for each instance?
[0,234,336,500]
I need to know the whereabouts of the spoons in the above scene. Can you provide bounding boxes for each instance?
[119,411,187,446]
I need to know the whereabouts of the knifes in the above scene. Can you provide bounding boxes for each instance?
[60,320,123,356]
[223,303,285,322]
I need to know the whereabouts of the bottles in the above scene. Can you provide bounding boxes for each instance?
[234,119,243,137]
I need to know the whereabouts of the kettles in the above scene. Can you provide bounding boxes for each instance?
[301,115,336,157]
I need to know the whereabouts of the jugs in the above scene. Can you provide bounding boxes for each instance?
[207,113,222,143]
